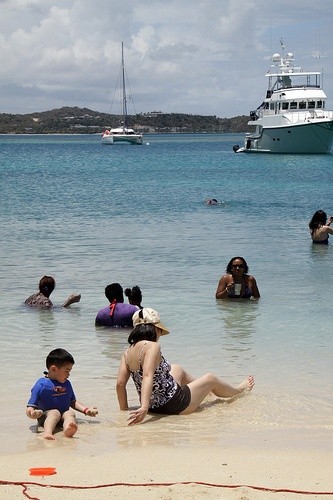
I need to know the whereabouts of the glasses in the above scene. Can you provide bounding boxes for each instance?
[232,264,244,268]
[157,327,163,333]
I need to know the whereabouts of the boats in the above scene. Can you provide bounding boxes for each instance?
[243,37,333,154]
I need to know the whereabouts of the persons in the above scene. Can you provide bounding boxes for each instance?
[116,308,255,426]
[24,276,81,312]
[215,257,260,302]
[26,347,98,440]
[95,283,140,328]
[308,210,333,245]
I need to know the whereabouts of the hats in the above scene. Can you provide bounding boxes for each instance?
[132,308,170,335]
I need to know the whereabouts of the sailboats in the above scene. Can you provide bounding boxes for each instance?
[101,40,143,145]
